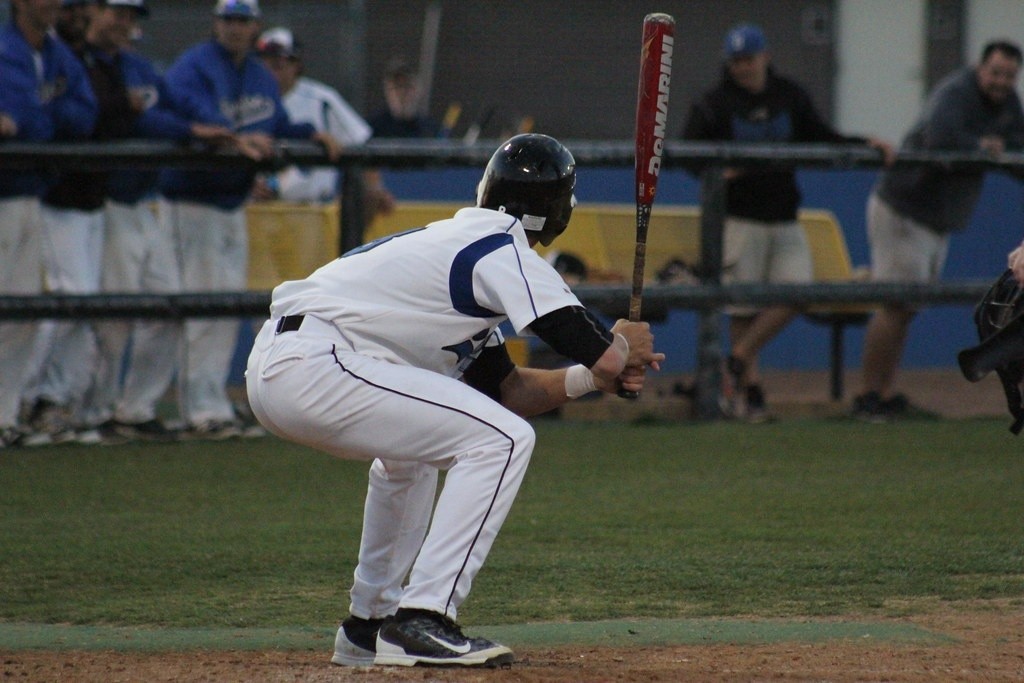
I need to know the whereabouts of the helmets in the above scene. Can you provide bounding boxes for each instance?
[477,133,579,247]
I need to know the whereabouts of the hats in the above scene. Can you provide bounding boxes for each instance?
[214,0,263,19]
[99,1,150,21]
[256,28,303,60]
[719,25,766,59]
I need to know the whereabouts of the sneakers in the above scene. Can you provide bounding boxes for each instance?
[329,615,379,666]
[373,611,516,668]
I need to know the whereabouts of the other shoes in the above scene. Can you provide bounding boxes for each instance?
[856,396,927,417]
[716,358,739,418]
[4,412,263,447]
[745,391,771,425]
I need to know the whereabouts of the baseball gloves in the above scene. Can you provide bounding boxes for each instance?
[954,265,1024,435]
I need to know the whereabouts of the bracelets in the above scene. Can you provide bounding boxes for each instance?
[565,364,598,399]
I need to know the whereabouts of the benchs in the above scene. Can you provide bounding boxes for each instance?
[246,207,875,401]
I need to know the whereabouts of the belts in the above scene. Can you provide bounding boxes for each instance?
[275,315,305,334]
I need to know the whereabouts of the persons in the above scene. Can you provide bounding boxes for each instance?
[683,23,895,422]
[524,249,586,421]
[165,0,341,443]
[849,42,1024,426]
[57,0,274,443]
[0,0,99,448]
[366,59,448,171]
[245,134,665,667]
[254,27,393,212]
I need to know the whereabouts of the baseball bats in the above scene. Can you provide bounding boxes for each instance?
[617,11,678,400]
